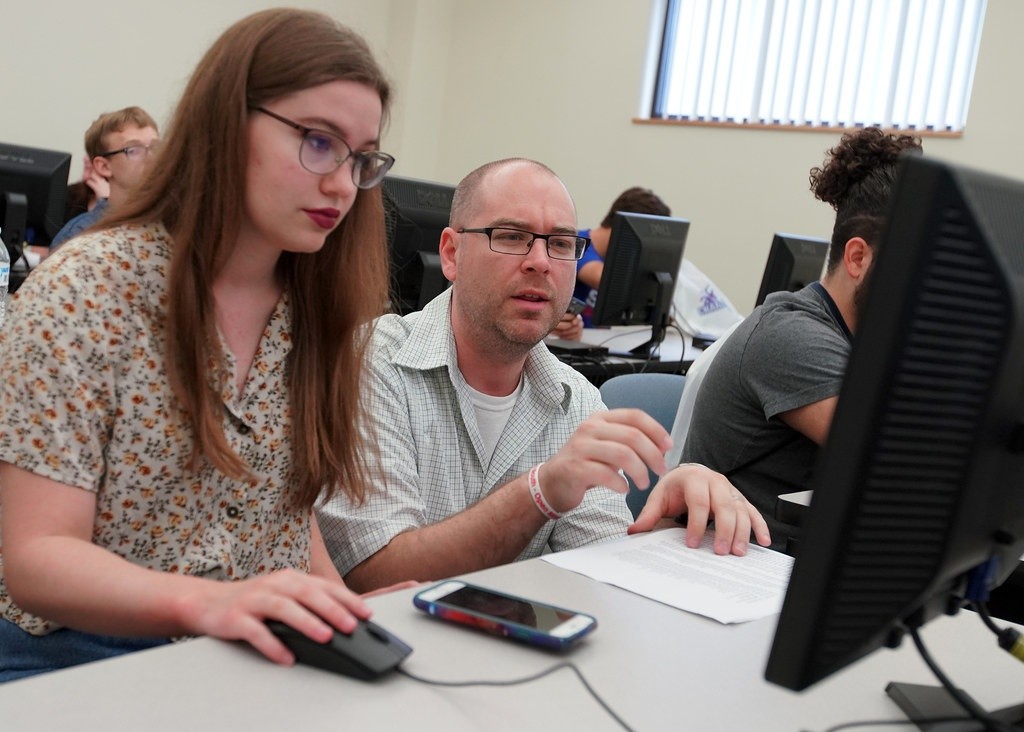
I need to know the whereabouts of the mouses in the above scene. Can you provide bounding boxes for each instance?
[264,613,414,683]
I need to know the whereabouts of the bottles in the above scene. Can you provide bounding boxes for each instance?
[0,227,10,329]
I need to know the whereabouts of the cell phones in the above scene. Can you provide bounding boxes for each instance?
[414,579,597,654]
[566,298,586,317]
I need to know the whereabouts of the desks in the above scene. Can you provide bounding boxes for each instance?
[0,528,1024,732]
[540,323,714,389]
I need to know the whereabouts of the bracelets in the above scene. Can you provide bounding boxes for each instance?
[528,462,565,519]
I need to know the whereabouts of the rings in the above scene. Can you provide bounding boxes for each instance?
[732,497,744,504]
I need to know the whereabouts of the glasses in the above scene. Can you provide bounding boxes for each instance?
[248,104,395,190]
[455,225,592,262]
[99,145,159,163]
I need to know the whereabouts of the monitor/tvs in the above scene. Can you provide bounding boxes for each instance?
[591,210,690,360]
[0,145,72,273]
[379,177,457,317]
[756,232,830,309]
[764,154,1024,732]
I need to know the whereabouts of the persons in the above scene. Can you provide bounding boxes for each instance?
[0,7,416,687]
[47,107,924,593]
